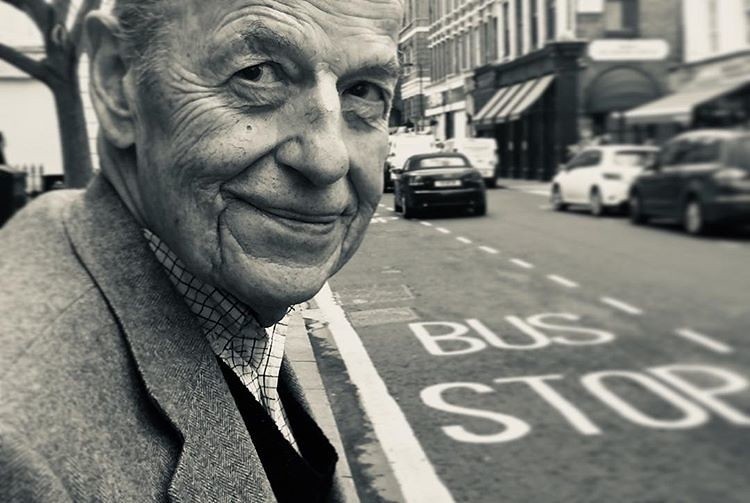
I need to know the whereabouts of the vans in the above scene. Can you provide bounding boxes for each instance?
[383,133,499,194]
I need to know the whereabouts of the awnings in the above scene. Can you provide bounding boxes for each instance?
[588,68,657,114]
[472,75,555,126]
[624,76,750,125]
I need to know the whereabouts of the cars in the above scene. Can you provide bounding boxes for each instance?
[390,152,488,220]
[550,144,661,216]
[626,126,750,238]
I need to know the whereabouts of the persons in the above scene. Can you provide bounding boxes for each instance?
[0,0,401,503]
